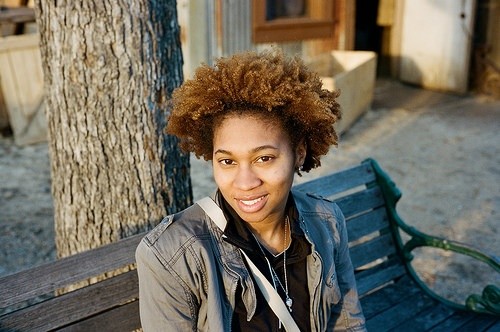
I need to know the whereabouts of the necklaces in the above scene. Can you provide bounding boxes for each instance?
[245,216,293,313]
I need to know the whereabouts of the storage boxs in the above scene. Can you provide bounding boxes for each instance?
[313,51,375,134]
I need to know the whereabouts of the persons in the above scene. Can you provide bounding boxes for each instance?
[134,48,368,332]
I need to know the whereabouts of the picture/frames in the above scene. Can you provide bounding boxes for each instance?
[250,1,334,41]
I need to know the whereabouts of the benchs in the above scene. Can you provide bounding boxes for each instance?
[0,157,499,332]
